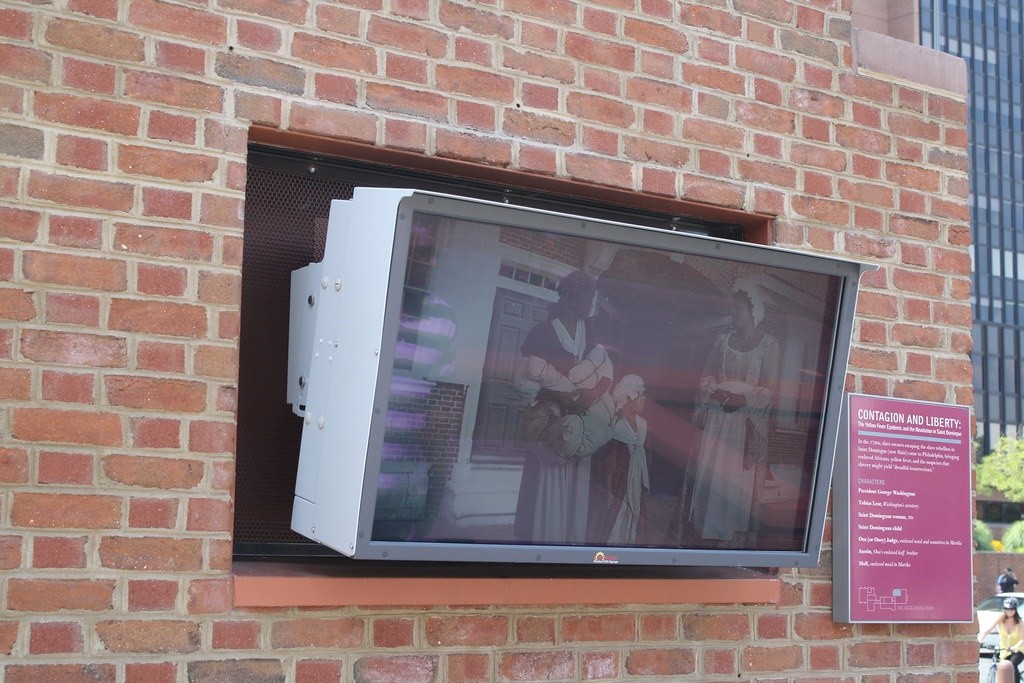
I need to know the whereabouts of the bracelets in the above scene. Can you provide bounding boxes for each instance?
[721,398,729,407]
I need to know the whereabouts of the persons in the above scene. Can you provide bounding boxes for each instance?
[512,271,613,543]
[979,598,1024,683]
[681,278,779,550]
[590,374,652,544]
[997,568,1019,592]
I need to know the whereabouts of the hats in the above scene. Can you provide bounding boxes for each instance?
[727,278,765,328]
[556,270,595,300]
[613,374,645,413]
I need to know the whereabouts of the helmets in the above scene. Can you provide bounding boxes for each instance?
[1003,597,1017,609]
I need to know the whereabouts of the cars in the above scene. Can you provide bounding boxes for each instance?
[974,592,1024,655]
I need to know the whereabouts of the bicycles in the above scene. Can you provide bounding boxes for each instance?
[979,643,1024,683]
